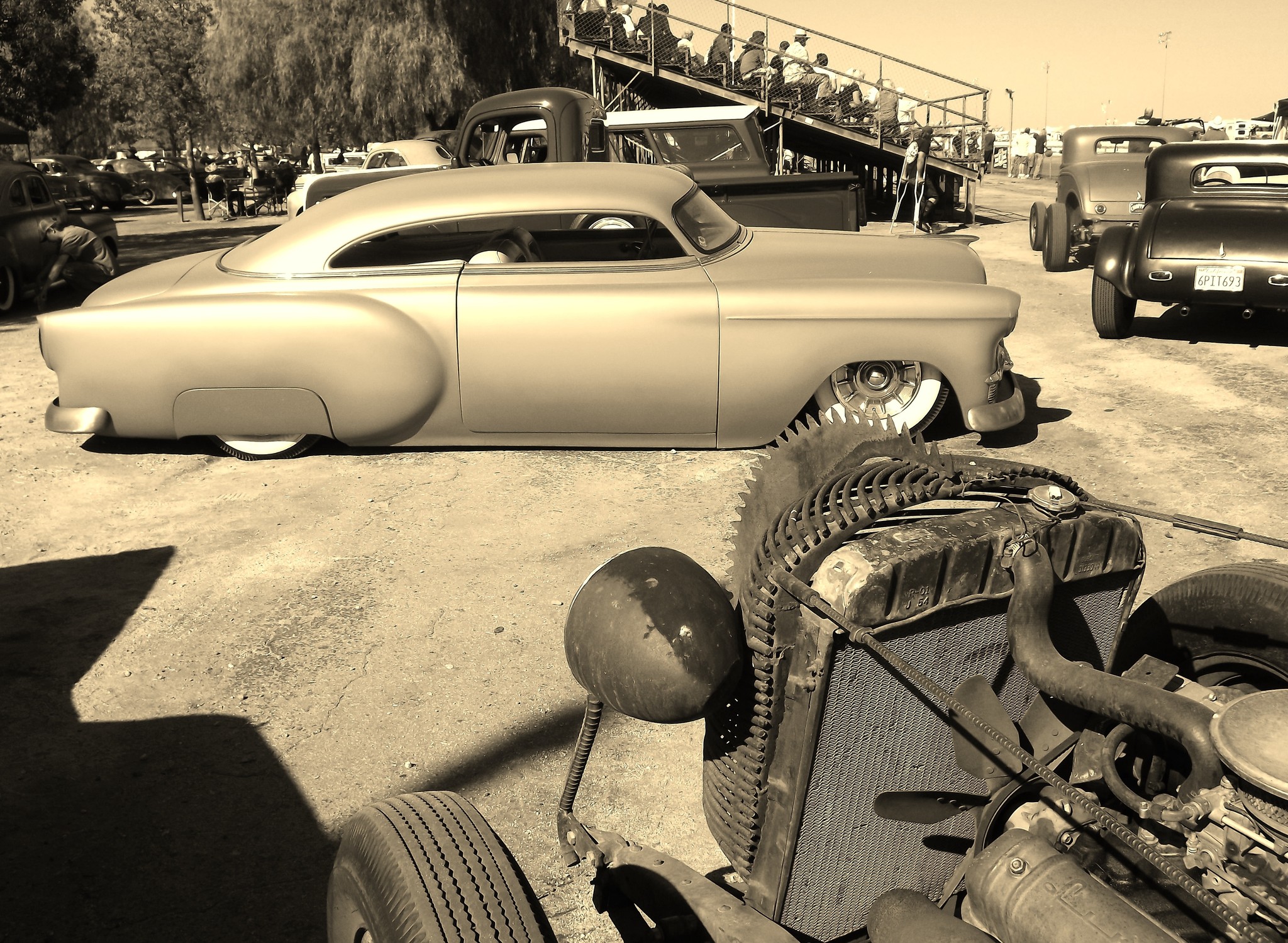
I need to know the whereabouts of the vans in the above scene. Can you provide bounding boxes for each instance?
[494,105,773,182]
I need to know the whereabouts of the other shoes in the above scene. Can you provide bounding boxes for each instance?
[1025,174,1030,178]
[913,218,933,233]
[1017,174,1025,179]
[1011,174,1015,178]
[1008,173,1012,178]
[1032,176,1040,180]
[932,224,947,234]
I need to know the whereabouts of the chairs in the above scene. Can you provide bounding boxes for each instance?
[475,238,528,262]
[205,166,295,221]
[491,227,544,262]
[533,146,547,161]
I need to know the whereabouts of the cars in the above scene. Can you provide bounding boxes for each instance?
[1029,125,1194,272]
[37,160,1027,465]
[324,403,1288,942]
[0,160,118,317]
[24,148,296,213]
[1091,141,1288,338]
[285,127,455,219]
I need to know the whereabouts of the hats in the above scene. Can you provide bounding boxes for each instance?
[793,28,811,39]
[622,5,632,11]
[749,31,766,43]
[988,127,993,131]
[923,125,933,134]
[38,217,58,243]
[1020,128,1024,132]
[655,4,669,14]
[747,31,765,42]
[817,53,828,66]
[897,87,906,94]
[684,28,695,36]
[128,146,140,151]
[204,162,219,173]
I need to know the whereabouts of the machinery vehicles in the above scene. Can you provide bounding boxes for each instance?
[449,86,868,233]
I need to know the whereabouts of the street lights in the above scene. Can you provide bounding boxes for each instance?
[1157,31,1173,120]
[1041,60,1050,131]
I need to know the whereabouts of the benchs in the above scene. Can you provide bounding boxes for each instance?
[558,13,954,157]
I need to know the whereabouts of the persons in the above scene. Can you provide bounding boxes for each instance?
[564,0,636,45]
[945,127,995,174]
[711,23,737,82]
[301,146,309,167]
[307,144,326,174]
[862,78,921,145]
[1008,127,1047,180]
[637,3,673,48]
[901,126,947,234]
[840,67,874,124]
[337,148,345,164]
[671,29,704,73]
[200,151,296,217]
[127,147,141,161]
[1200,115,1229,141]
[1128,107,1165,153]
[776,146,794,175]
[103,151,117,172]
[32,218,117,303]
[735,31,778,92]
[771,28,837,112]
